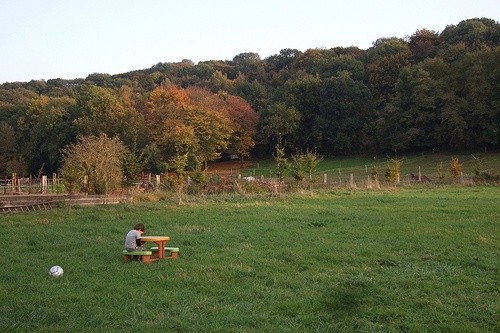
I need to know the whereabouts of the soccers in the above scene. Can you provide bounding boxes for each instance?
[49,266,63,277]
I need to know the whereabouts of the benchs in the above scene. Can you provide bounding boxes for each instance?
[123,248,153,265]
[149,246,180,261]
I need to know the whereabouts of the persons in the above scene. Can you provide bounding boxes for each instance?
[125,223,146,260]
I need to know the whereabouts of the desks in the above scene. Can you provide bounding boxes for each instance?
[138,236,170,261]
